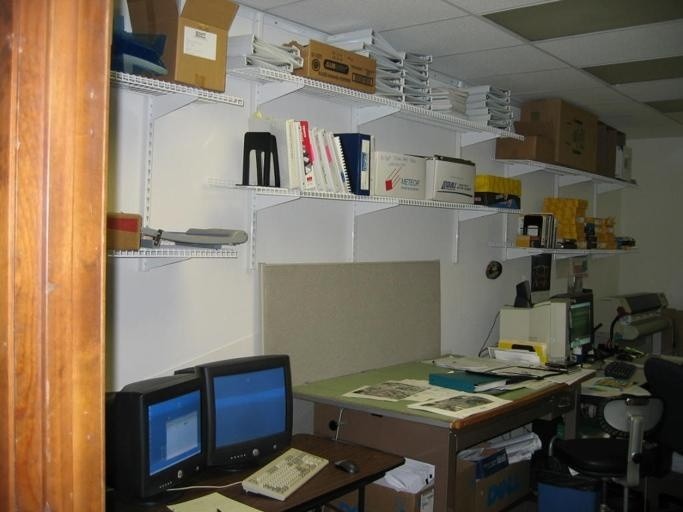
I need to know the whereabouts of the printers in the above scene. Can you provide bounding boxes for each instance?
[598,293,674,353]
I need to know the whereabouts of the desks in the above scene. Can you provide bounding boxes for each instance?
[568,350,683,396]
[291,355,596,512]
[106,432,404,512]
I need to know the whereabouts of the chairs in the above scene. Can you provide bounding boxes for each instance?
[552,359,683,512]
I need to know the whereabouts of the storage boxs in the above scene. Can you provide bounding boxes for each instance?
[496,97,630,179]
[284,40,376,91]
[456,423,532,512]
[328,483,435,512]
[375,150,521,210]
[128,0,241,92]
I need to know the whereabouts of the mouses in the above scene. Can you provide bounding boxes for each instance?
[334,460,359,474]
[616,355,633,362]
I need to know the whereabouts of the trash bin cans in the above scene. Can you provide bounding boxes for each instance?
[536,464,603,512]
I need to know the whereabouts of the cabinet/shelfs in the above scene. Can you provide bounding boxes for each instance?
[211,68,524,270]
[493,159,639,262]
[106,70,243,271]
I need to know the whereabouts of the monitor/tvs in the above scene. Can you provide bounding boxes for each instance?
[174,354,293,473]
[498,292,595,365]
[105,373,205,506]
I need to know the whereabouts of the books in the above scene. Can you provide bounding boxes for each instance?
[517,211,557,249]
[260,110,371,199]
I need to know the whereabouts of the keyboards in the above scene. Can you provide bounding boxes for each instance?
[605,361,638,379]
[242,448,329,502]
